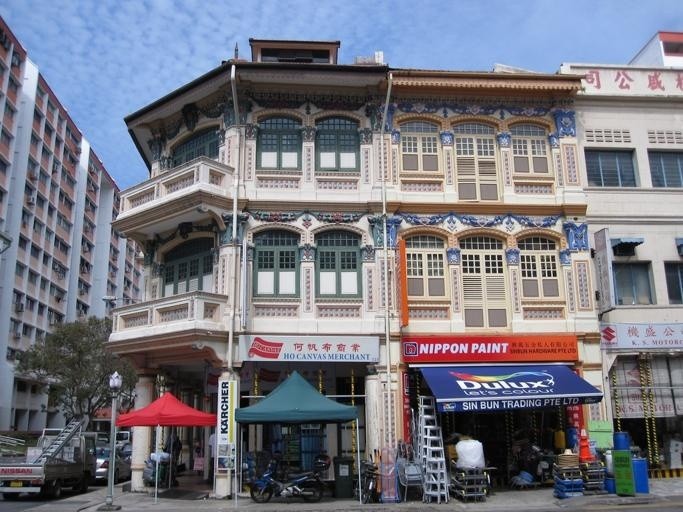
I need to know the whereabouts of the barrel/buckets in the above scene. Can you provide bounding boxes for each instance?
[632,459,649,494]
[604,475,616,494]
[613,432,630,450]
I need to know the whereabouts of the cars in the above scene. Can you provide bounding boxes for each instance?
[95,430,131,484]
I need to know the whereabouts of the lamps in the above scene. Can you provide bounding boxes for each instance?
[101,295,142,304]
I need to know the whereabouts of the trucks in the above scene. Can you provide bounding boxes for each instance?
[0,417,97,500]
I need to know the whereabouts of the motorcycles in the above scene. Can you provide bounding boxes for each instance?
[248,448,330,503]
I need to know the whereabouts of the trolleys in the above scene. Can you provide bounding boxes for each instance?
[449,460,487,504]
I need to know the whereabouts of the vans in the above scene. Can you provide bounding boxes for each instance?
[41,427,63,440]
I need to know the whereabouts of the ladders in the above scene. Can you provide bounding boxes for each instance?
[416,396,450,504]
[33,417,85,463]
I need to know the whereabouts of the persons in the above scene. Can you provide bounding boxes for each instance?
[196,443,201,457]
[173,437,182,466]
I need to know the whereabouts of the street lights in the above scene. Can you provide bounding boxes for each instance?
[97,370,123,511]
[100,295,141,302]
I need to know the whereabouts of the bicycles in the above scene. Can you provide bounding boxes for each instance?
[354,454,379,503]
